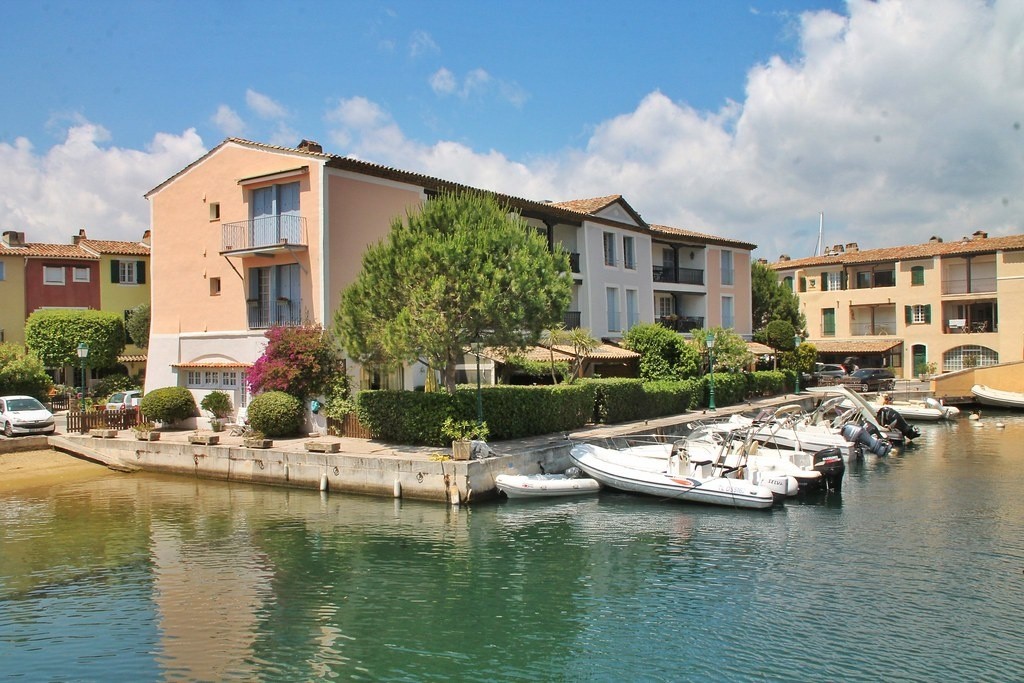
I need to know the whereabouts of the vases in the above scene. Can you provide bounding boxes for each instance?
[247,302,259,308]
[304,441,341,454]
[277,300,288,306]
[188,434,219,445]
[89,429,117,439]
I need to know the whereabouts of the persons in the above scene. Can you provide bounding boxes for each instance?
[853,364,859,373]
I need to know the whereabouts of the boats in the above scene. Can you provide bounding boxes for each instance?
[495,473,601,499]
[568,384,960,508]
[971,384,1024,407]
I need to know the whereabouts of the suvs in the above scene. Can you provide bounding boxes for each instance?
[803,364,846,381]
[105,390,143,419]
[850,367,895,392]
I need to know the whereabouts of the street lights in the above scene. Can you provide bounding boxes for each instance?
[706,332,716,411]
[469,332,485,439]
[794,336,800,395]
[76,342,88,435]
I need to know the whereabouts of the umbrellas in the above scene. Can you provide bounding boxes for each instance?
[426,355,438,393]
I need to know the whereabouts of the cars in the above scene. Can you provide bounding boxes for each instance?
[0,395,56,437]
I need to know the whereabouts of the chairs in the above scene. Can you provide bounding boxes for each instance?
[960,321,988,333]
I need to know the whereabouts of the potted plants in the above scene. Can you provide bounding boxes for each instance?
[243,430,273,449]
[441,416,489,461]
[917,362,938,382]
[200,389,234,433]
[129,421,160,441]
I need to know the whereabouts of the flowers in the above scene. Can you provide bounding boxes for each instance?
[246,298,260,302]
[276,296,289,301]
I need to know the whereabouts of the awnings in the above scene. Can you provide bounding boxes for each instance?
[602,336,625,345]
[461,345,575,363]
[800,339,903,352]
[538,338,642,358]
[685,339,784,353]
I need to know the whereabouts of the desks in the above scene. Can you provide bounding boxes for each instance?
[970,322,982,332]
[864,323,888,335]
